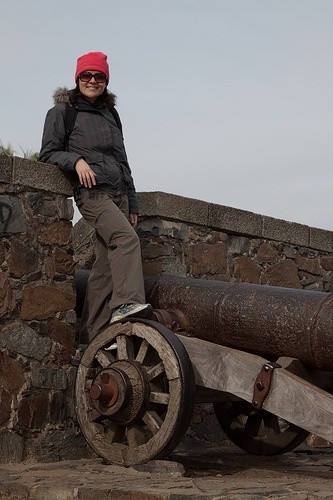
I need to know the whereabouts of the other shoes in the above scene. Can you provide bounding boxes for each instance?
[109,303,153,323]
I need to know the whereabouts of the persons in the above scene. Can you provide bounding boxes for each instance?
[36,52,152,351]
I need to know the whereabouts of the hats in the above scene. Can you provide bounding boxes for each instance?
[74,51,110,83]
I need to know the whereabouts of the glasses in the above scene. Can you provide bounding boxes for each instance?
[77,71,108,83]
